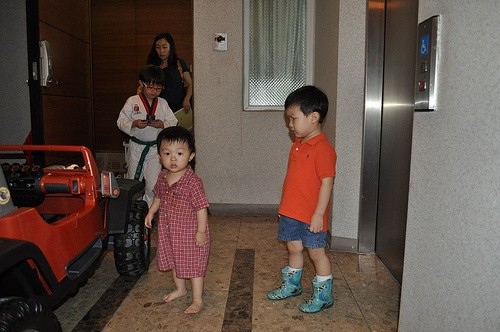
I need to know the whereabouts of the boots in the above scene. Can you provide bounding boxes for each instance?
[268,267,303,300]
[299,275,334,314]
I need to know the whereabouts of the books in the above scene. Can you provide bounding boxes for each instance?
[174,106,193,133]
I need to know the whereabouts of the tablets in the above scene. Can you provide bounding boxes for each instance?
[174,107,193,133]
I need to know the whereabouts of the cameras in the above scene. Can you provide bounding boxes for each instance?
[146,114,156,123]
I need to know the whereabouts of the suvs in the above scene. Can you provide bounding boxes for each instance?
[0,144,151,332]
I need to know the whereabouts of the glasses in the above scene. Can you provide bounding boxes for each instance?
[142,83,163,92]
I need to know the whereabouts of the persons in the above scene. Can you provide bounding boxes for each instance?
[267,85,337,313]
[136,33,193,135]
[145,126,211,314]
[117,65,178,227]
